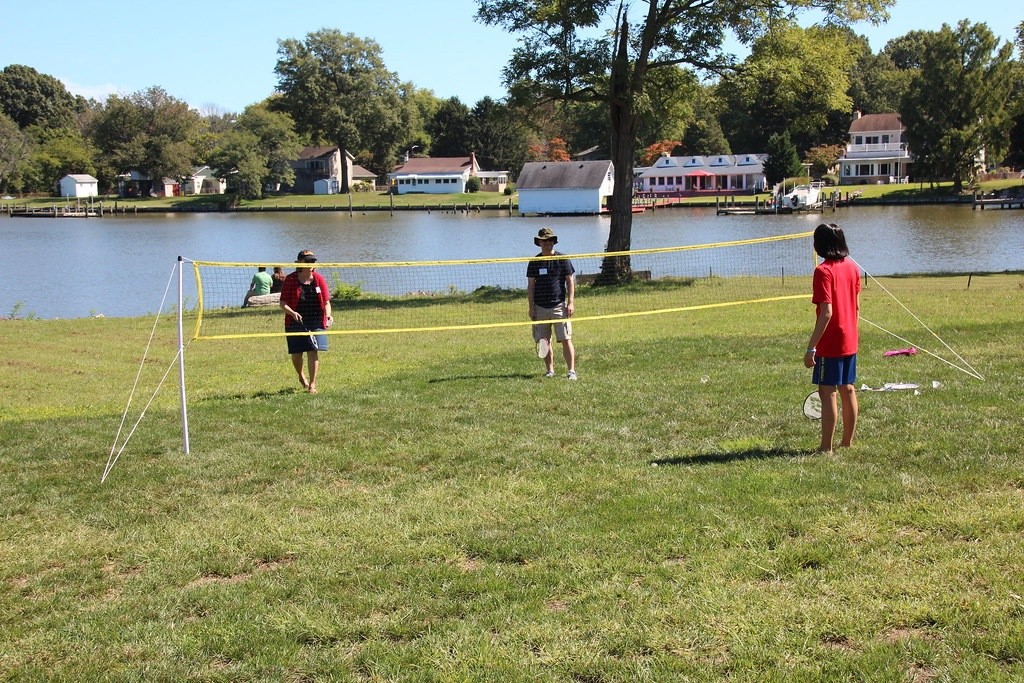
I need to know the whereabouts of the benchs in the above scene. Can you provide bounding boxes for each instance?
[575,270,652,285]
[248,292,281,307]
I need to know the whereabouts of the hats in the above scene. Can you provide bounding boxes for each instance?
[297,250,317,261]
[533,228,558,246]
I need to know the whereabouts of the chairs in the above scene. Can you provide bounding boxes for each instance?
[901,175,909,184]
[889,176,897,184]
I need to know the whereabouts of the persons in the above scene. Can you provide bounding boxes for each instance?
[693,183,696,190]
[804,222,861,457]
[526,228,577,381]
[241,267,286,308]
[279,249,333,395]
[791,196,799,207]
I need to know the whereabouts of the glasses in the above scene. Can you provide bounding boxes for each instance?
[301,259,315,263]
[540,238,554,242]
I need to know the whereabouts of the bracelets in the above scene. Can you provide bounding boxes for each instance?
[807,347,816,353]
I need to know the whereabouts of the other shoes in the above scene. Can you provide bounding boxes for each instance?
[567,370,577,380]
[309,386,316,394]
[546,370,554,377]
[241,305,246,308]
[299,378,309,388]
